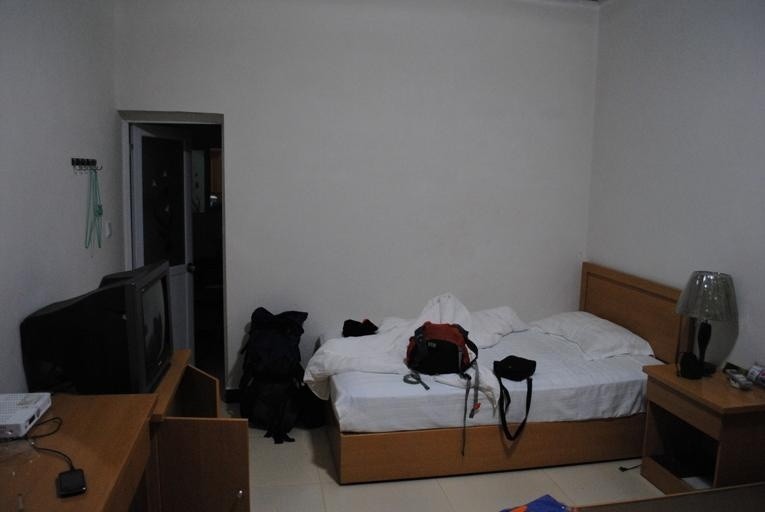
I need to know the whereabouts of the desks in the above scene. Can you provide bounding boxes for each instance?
[0,395,160,511]
[568,482,764,511]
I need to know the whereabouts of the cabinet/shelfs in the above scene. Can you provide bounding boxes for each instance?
[152,348,250,512]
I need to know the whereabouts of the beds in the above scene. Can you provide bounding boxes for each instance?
[319,261,681,485]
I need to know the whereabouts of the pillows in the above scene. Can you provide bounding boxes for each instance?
[529,311,653,362]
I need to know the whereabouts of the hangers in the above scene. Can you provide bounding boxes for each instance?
[72,157,103,174]
[95,170,104,248]
[85,171,95,249]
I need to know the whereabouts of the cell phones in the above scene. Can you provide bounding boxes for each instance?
[58,469,88,498]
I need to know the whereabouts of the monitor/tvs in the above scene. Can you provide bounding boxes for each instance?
[19,260,174,394]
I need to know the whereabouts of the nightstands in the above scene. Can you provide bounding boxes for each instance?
[639,363,764,495]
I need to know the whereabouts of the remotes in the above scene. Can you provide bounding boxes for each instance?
[725,367,753,390]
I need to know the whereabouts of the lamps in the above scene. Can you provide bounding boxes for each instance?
[674,271,739,380]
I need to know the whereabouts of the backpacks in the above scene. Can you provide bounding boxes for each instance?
[402,321,478,456]
[275,362,332,431]
[238,307,308,433]
